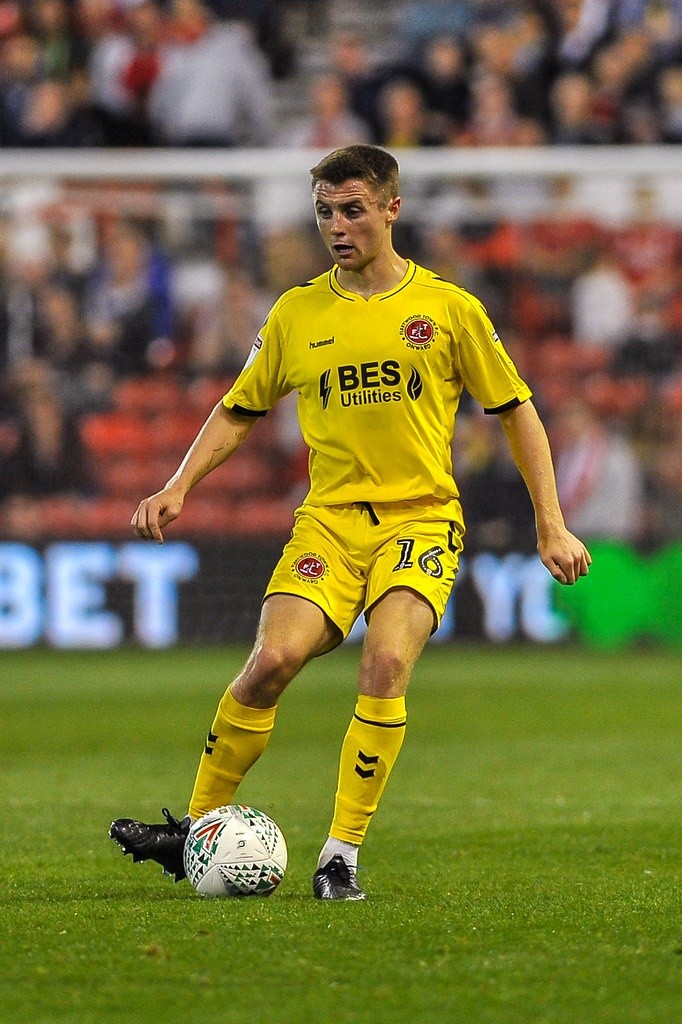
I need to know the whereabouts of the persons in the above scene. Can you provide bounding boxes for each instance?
[1,1,681,547]
[109,146,591,902]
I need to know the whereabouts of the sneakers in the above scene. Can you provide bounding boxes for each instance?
[109,809,192,882]
[313,852,368,900]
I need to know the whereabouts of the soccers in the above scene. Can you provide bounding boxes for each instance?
[182,803,290,899]
